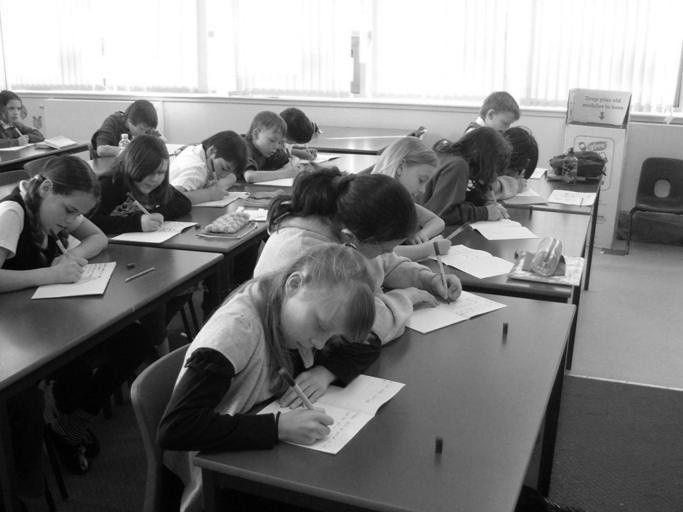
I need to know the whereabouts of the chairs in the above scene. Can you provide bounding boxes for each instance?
[622,156,681,252]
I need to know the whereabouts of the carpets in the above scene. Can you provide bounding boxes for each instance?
[0,367,681,512]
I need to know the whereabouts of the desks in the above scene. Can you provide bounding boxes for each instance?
[1,126,601,512]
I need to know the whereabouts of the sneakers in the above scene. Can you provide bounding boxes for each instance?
[36,376,102,461]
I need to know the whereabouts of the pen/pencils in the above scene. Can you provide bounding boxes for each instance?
[285,148,294,169]
[444,220,472,240]
[48,227,71,258]
[10,122,23,136]
[124,267,155,283]
[432,241,451,304]
[278,367,316,410]
[211,158,219,184]
[304,143,311,154]
[519,158,531,178]
[126,192,150,215]
[488,185,498,204]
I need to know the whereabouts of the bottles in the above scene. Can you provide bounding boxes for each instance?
[118,133,132,155]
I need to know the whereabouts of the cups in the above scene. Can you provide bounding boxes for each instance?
[531,236,566,276]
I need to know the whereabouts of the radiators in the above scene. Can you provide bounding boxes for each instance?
[39,97,164,139]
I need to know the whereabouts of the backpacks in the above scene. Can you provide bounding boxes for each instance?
[548,149,606,178]
